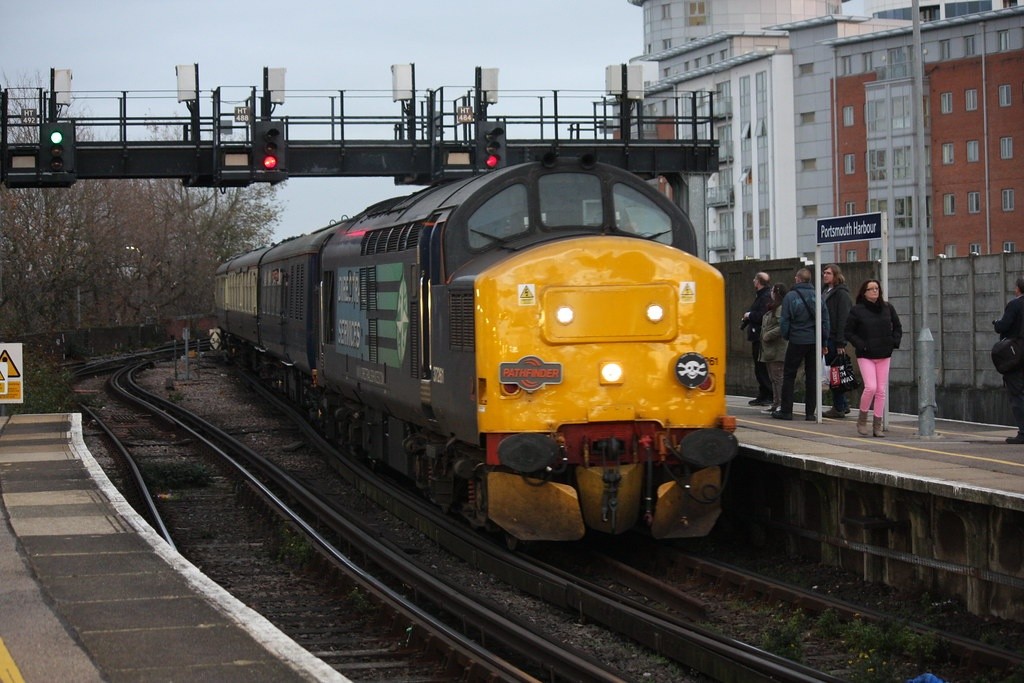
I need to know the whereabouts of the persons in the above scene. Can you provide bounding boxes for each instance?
[741,263,852,421]
[991,279,1024,444]
[843,279,902,438]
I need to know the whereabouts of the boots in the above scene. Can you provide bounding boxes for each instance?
[856,409,870,437]
[876,417,887,438]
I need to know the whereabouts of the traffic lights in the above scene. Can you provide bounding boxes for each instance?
[39,121,76,172]
[475,120,506,174]
[252,120,287,171]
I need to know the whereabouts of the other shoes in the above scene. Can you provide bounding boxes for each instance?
[748,393,772,405]
[1006,433,1024,444]
[804,410,815,419]
[761,405,780,415]
[773,410,793,420]
[822,408,851,420]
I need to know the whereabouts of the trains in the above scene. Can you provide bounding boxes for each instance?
[210,141,742,555]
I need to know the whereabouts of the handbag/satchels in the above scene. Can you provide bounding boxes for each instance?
[991,335,1024,375]
[830,354,858,392]
[820,355,832,395]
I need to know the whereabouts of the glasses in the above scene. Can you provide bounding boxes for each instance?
[753,278,756,283]
[865,287,880,291]
[822,272,833,275]
